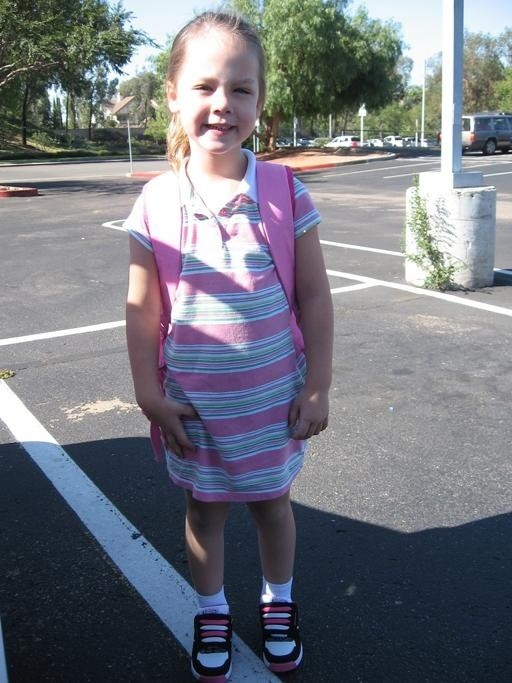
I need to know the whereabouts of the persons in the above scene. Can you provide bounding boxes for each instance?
[122,10,334,683]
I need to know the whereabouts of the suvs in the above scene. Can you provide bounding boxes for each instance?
[462,111,512,154]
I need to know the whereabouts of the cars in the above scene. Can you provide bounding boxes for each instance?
[276,132,439,148]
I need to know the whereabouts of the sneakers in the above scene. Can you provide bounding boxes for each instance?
[259,585,303,672]
[190,604,233,683]
